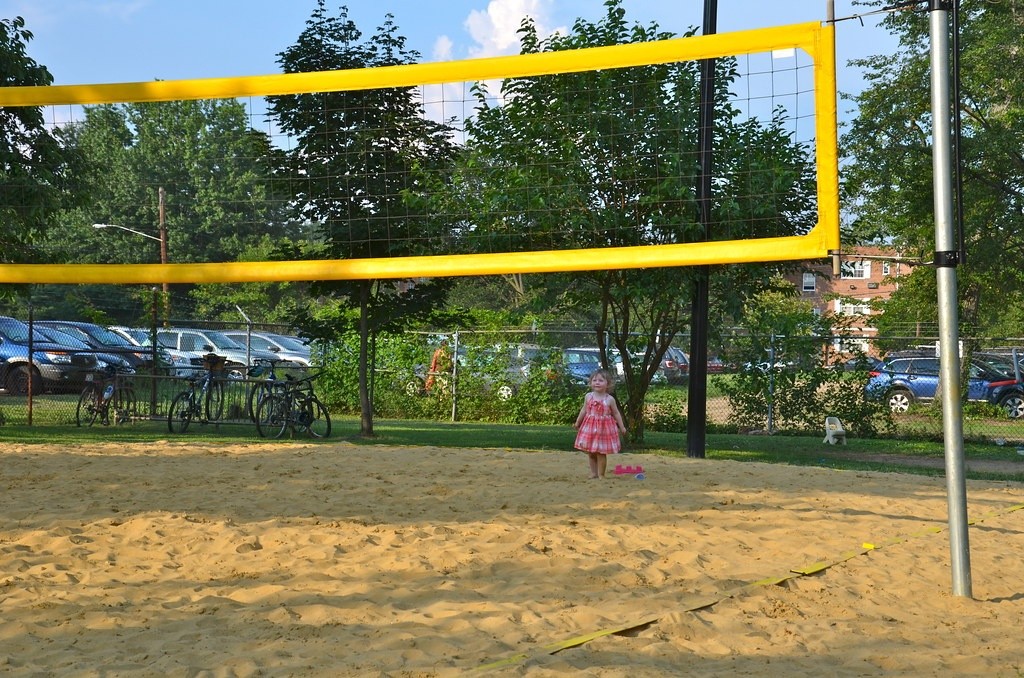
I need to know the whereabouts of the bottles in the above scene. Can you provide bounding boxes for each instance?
[103,383,113,398]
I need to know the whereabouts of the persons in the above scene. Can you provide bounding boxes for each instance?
[573,369,627,481]
[424,339,451,391]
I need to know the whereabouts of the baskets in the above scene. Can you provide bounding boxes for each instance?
[203,353,226,371]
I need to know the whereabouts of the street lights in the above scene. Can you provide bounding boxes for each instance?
[93,222,173,326]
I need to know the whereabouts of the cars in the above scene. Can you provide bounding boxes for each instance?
[398,340,808,402]
[24,324,136,384]
[107,325,174,374]
[872,350,1024,391]
[861,357,1024,420]
[825,356,882,373]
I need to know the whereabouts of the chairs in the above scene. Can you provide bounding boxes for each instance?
[823,417,846,445]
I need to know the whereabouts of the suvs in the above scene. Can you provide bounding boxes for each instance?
[0,315,98,396]
[140,328,283,382]
[219,331,327,375]
[22,321,174,380]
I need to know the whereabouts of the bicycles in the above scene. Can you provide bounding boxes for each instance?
[246,357,333,441]
[75,357,137,429]
[167,355,233,434]
